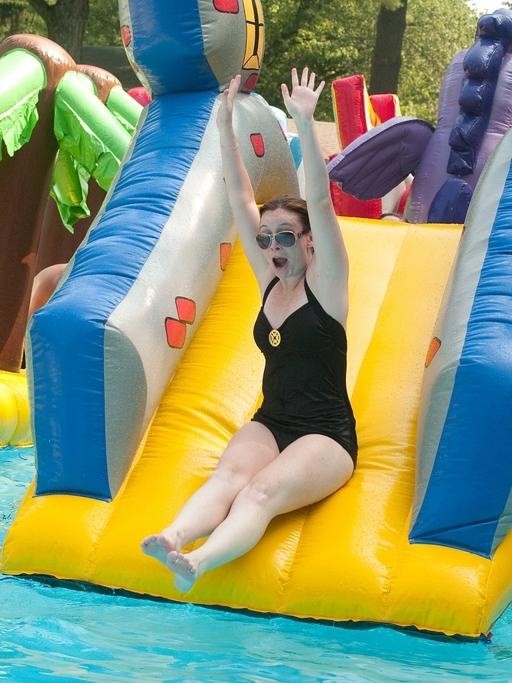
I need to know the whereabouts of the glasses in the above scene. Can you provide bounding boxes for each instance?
[255,231,309,250]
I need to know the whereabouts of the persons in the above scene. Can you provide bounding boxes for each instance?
[138,66,360,595]
